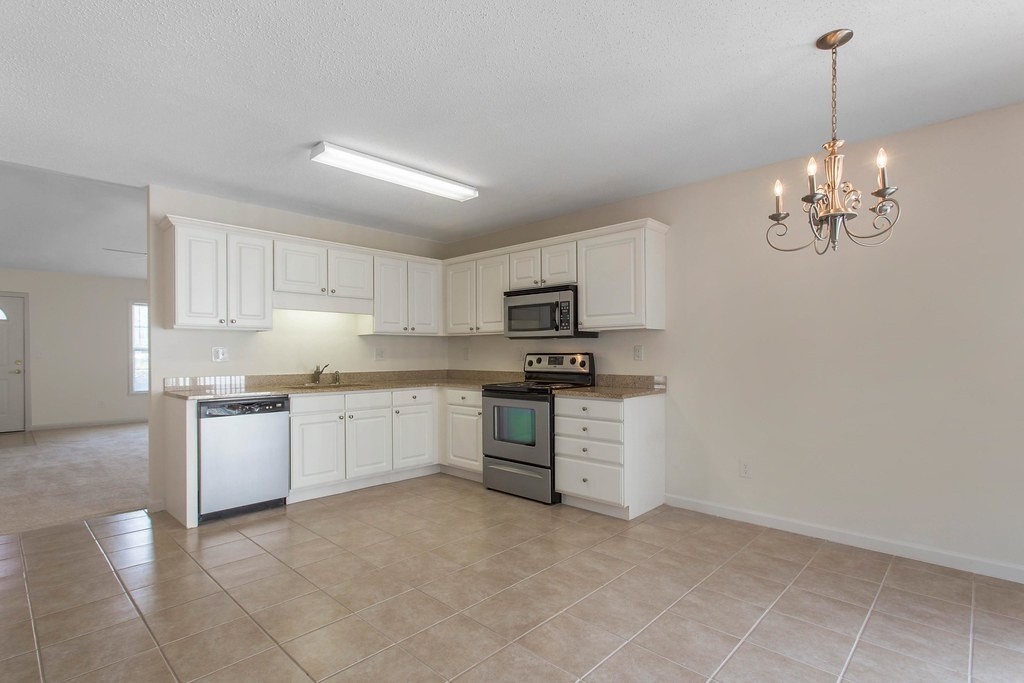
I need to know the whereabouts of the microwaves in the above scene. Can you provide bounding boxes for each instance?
[504,285,599,340]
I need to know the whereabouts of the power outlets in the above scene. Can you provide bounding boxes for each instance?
[463,347,471,360]
[515,348,524,361]
[634,344,644,360]
[212,346,228,362]
[374,348,385,360]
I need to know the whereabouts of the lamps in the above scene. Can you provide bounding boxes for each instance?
[765,28,901,255]
[309,140,479,203]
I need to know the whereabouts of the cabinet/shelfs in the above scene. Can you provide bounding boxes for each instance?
[393,387,441,484]
[159,220,274,332]
[272,239,374,315]
[553,393,668,520]
[439,386,483,483]
[509,240,578,292]
[286,389,393,505]
[358,252,444,336]
[579,226,667,331]
[445,254,509,336]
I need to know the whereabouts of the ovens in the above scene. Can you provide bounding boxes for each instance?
[481,390,562,505]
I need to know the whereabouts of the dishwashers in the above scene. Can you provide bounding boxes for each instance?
[198,394,291,526]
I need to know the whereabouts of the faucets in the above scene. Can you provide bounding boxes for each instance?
[313,363,330,384]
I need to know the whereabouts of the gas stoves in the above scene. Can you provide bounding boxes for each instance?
[482,351,595,395]
